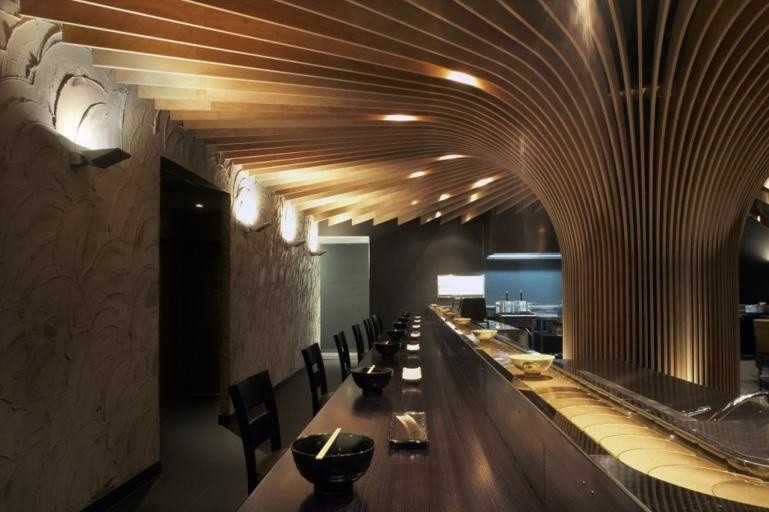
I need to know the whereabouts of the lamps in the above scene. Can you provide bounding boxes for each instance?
[363,318,375,352]
[333,330,353,383]
[351,323,366,365]
[228,369,292,496]
[302,342,339,418]
[371,314,380,342]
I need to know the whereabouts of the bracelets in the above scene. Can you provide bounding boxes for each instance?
[472,329,498,342]
[374,342,401,357]
[350,367,393,394]
[453,317,471,325]
[386,328,406,341]
[397,317,409,322]
[508,354,556,378]
[401,314,410,317]
[289,432,376,492]
[392,322,407,328]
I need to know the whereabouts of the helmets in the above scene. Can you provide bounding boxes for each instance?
[414,316,421,319]
[388,410,428,444]
[406,344,420,351]
[401,366,422,382]
[414,320,421,323]
[410,332,421,338]
[412,324,421,328]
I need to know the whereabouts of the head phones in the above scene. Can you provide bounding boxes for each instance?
[367,364,375,374]
[315,426,342,460]
